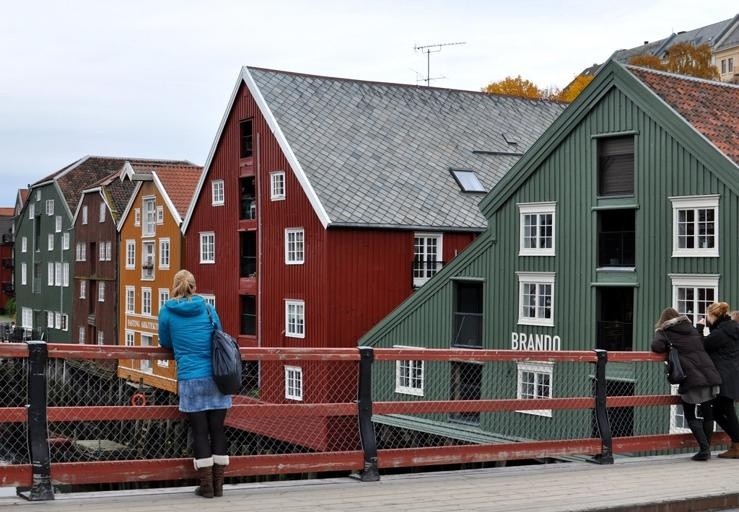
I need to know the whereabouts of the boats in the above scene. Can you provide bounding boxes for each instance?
[18,422,137,462]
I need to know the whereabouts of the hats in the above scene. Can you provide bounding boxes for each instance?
[660,306,679,320]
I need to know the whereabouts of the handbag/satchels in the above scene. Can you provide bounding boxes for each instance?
[204,303,242,395]
[662,329,686,384]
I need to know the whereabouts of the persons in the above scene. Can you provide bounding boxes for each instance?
[156,269,233,498]
[650,307,724,461]
[696,301,738,459]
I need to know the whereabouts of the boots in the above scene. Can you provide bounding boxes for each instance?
[717,441,739,459]
[212,454,229,497]
[193,455,214,498]
[688,418,714,461]
